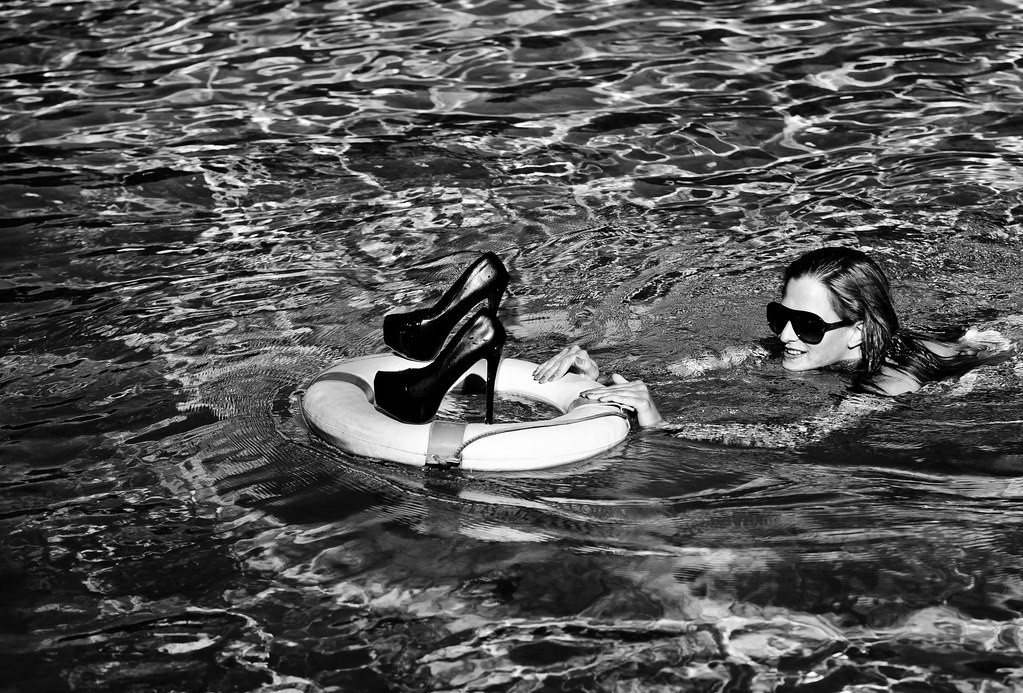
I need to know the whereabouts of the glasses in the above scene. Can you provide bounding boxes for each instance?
[767,302,854,345]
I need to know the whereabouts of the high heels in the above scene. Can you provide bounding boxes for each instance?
[374,307,507,425]
[383,251,509,361]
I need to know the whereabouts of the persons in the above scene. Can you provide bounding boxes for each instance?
[531,247,959,429]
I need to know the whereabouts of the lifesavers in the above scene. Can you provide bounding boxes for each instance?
[290,341,635,470]
